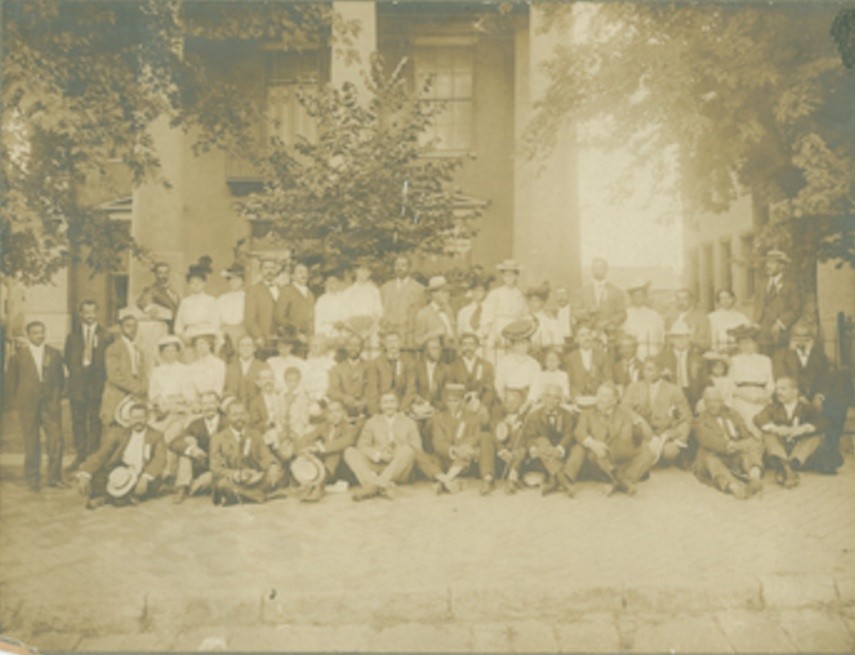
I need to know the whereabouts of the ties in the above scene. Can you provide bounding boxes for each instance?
[800,345,806,356]
[768,280,776,298]
[87,328,91,361]
[721,414,731,439]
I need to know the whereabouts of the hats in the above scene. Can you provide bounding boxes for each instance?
[497,259,524,271]
[414,327,446,348]
[502,319,536,340]
[114,394,153,427]
[226,263,244,279]
[182,320,223,350]
[158,335,184,356]
[667,321,692,337]
[765,251,792,263]
[526,281,550,300]
[290,454,326,485]
[424,276,454,293]
[105,468,136,497]
[726,324,761,338]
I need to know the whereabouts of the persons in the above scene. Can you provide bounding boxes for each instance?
[62,250,847,510]
[3,321,65,491]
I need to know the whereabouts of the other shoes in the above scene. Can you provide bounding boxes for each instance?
[25,455,844,508]
